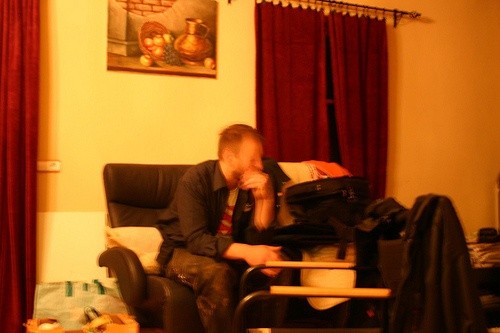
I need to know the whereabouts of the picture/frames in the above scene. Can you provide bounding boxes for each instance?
[107,0,217,78]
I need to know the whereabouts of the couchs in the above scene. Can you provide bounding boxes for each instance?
[100,164,204,333]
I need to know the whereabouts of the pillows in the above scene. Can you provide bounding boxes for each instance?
[105,227,164,275]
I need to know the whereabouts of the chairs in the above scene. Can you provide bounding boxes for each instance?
[231,196,448,333]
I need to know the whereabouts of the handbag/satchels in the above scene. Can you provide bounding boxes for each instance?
[284,175,369,205]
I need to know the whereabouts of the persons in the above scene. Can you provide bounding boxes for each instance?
[151,124,292,333]
